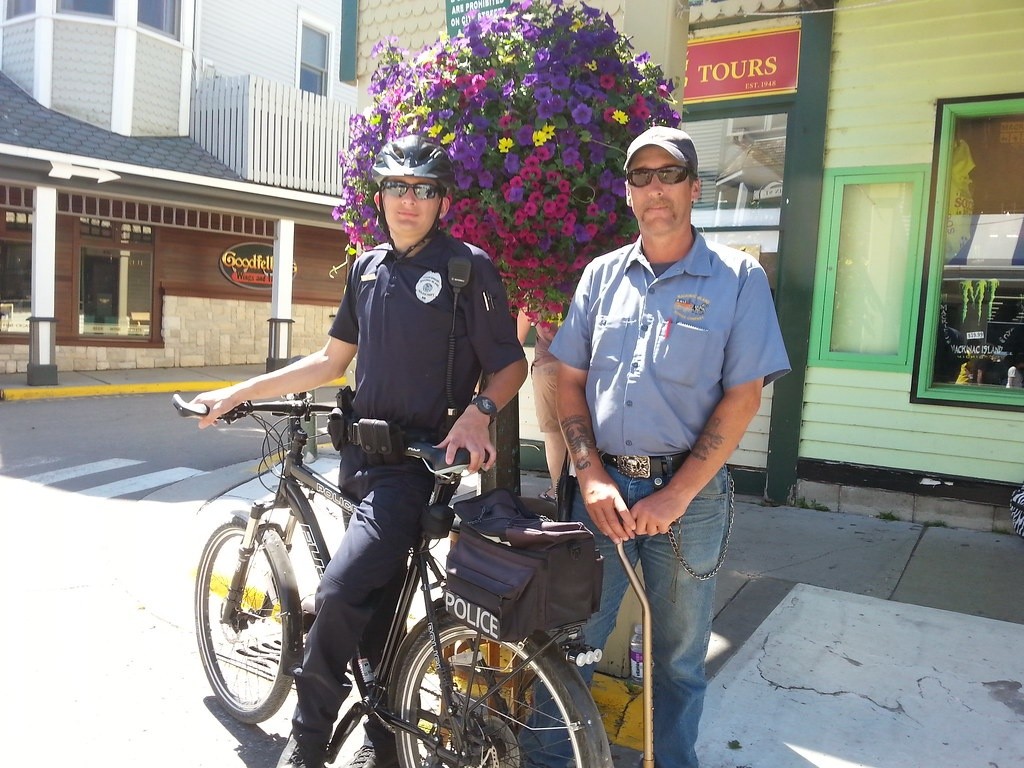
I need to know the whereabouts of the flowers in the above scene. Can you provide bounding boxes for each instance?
[330,1,689,336]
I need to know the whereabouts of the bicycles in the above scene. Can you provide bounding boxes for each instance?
[170,392,615,768]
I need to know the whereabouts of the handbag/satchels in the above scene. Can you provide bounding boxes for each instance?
[444,486,604,642]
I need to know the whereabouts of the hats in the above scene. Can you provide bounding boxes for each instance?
[623,126,699,176]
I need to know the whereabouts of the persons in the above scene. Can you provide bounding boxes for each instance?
[188,133,527,767]
[514,126,793,768]
[1005,352,1023,389]
[954,338,985,386]
[512,305,564,502]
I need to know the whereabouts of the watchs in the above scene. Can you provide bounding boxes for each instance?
[468,395,498,427]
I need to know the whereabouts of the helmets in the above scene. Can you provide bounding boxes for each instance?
[371,134,456,197]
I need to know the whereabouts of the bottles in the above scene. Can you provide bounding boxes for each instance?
[630,625,644,682]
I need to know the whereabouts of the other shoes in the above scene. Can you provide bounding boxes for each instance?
[342,746,376,768]
[284,743,318,768]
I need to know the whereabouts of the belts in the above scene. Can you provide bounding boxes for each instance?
[595,449,691,478]
[344,418,441,450]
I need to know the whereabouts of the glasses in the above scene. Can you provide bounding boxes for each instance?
[380,181,441,200]
[626,165,693,187]
